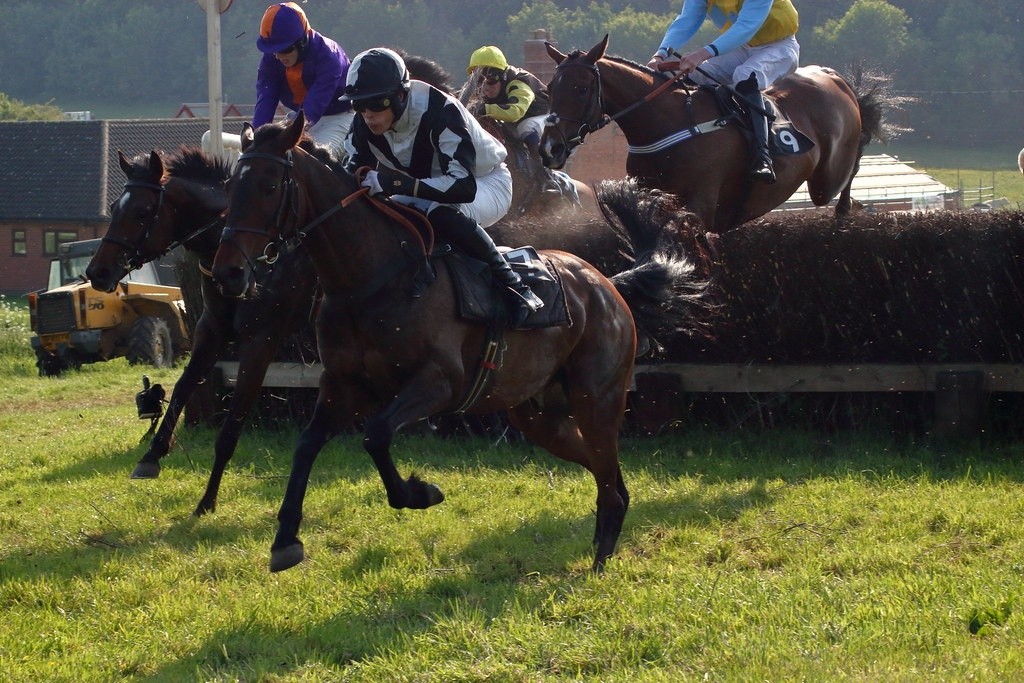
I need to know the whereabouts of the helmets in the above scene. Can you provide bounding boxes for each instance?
[338,47,410,101]
[465,45,508,75]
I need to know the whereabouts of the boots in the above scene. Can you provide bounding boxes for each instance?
[457,219,545,330]
[738,93,777,184]
[529,144,562,207]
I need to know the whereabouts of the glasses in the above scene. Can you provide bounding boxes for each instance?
[478,67,507,85]
[350,94,397,112]
[279,45,296,54]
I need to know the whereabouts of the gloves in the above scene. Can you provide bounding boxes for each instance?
[361,169,383,196]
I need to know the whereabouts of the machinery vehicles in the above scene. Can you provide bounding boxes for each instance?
[25,237,192,374]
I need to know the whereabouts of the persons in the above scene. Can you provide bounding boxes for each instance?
[251,2,354,165]
[466,45,550,166]
[341,46,547,327]
[645,0,801,183]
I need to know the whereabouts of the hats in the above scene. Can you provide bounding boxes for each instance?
[257,2,311,52]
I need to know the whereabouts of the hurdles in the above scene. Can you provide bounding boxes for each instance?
[181,196,1024,436]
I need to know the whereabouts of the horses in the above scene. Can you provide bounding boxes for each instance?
[87,33,919,572]
